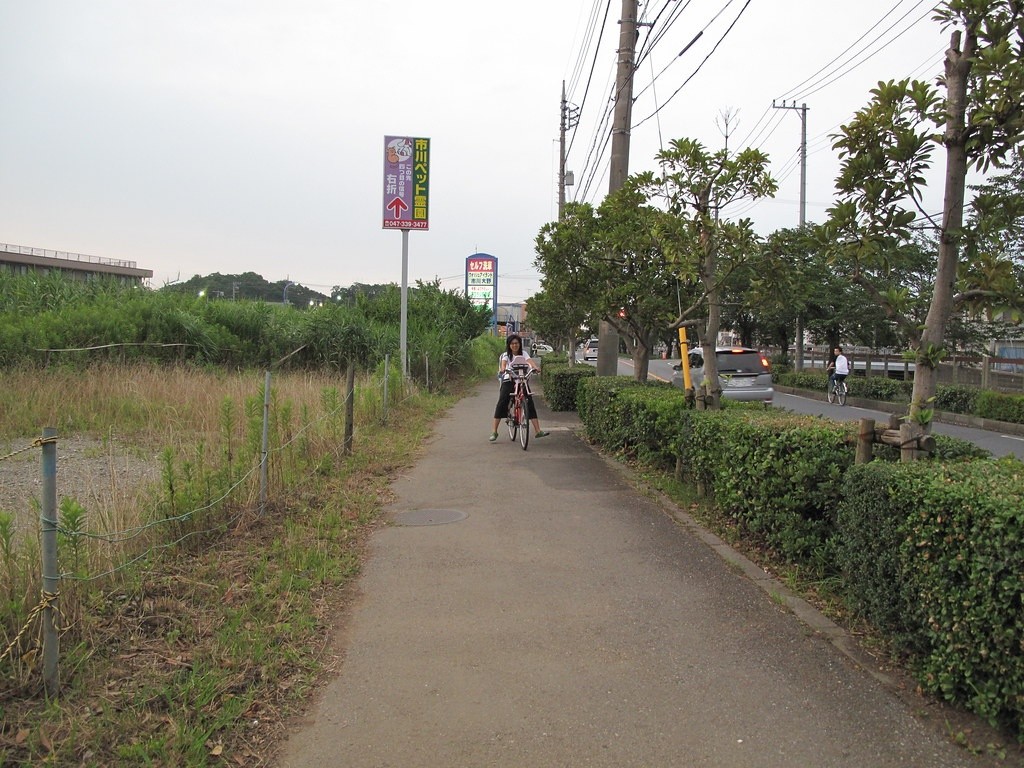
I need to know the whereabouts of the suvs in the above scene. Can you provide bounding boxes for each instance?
[669,344,774,409]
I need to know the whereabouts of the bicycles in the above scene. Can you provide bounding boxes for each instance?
[498,366,540,450]
[826,366,849,406]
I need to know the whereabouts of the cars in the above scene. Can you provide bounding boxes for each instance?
[537,345,554,356]
[584,339,599,362]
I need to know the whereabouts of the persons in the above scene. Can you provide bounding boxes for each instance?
[826,346,849,394]
[532,342,537,355]
[489,335,550,441]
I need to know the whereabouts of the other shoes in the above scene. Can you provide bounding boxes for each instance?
[535,431,549,438]
[489,432,498,441]
